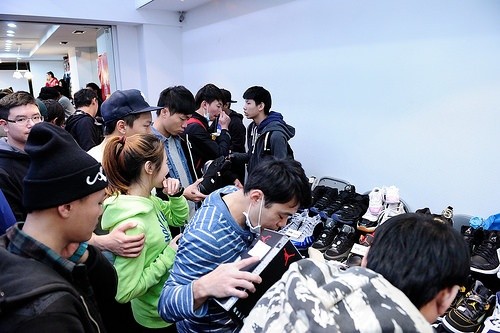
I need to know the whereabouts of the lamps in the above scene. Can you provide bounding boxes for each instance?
[13,60,23,79]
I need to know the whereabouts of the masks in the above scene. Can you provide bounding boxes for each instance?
[203,108,210,121]
[242,204,262,233]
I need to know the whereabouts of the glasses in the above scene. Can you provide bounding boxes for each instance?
[7,116,44,126]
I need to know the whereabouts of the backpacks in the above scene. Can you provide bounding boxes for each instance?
[267,130,294,161]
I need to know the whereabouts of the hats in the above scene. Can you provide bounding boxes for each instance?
[22,122,108,210]
[100,89,164,123]
[220,89,237,103]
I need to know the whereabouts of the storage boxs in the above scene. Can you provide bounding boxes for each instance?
[212,229,304,328]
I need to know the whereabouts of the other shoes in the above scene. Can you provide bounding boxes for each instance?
[197,155,232,195]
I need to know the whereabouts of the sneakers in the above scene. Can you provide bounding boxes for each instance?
[278,176,499,333]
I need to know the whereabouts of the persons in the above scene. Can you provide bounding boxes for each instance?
[180,83,232,183]
[40,99,65,128]
[158,156,311,333]
[239,212,472,333]
[209,88,247,154]
[243,86,295,173]
[100,134,190,333]
[66,88,102,152]
[45,71,60,88]
[86,88,165,258]
[0,91,45,229]
[150,84,208,234]
[0,122,118,333]
[1,82,102,123]
